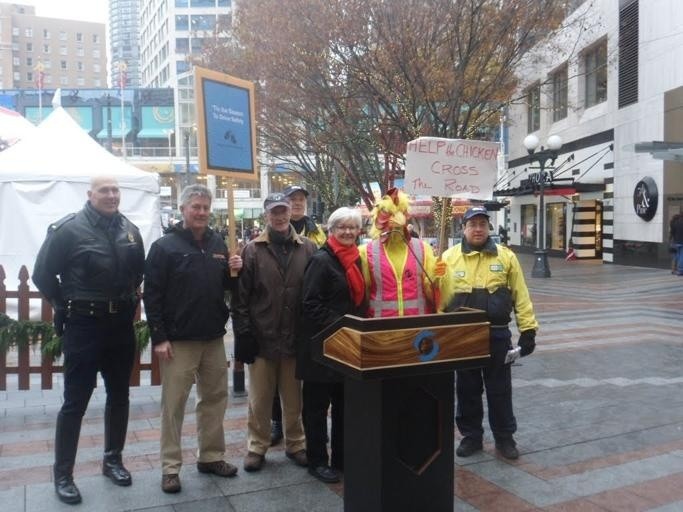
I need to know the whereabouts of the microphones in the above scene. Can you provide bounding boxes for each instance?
[382,231,437,313]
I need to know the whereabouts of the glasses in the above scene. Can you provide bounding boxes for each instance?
[335,224,358,232]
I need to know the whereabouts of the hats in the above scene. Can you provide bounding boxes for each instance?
[264,193,291,212]
[285,186,310,198]
[465,207,490,220]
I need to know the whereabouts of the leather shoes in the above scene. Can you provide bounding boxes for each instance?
[102,454,132,487]
[53,467,82,505]
[308,464,339,483]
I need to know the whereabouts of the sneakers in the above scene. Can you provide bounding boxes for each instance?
[286,449,310,468]
[244,452,267,471]
[162,474,181,493]
[271,422,282,443]
[456,435,483,457]
[197,462,238,476]
[496,441,519,460]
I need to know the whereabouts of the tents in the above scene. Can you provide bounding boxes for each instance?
[0,88,163,321]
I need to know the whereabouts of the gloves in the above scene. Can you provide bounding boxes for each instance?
[50,297,69,337]
[237,334,258,364]
[519,329,536,357]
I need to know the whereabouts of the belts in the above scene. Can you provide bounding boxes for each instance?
[67,300,134,314]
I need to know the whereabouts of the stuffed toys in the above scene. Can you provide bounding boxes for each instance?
[358,188,448,323]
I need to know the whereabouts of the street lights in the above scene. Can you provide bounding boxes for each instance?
[28,60,52,123]
[108,54,129,164]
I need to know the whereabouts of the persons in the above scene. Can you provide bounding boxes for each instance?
[215,216,262,241]
[407,223,419,238]
[437,205,540,460]
[670,212,683,276]
[230,192,320,473]
[30,172,146,506]
[312,216,326,232]
[143,183,243,495]
[499,226,508,245]
[258,184,329,448]
[293,205,366,484]
